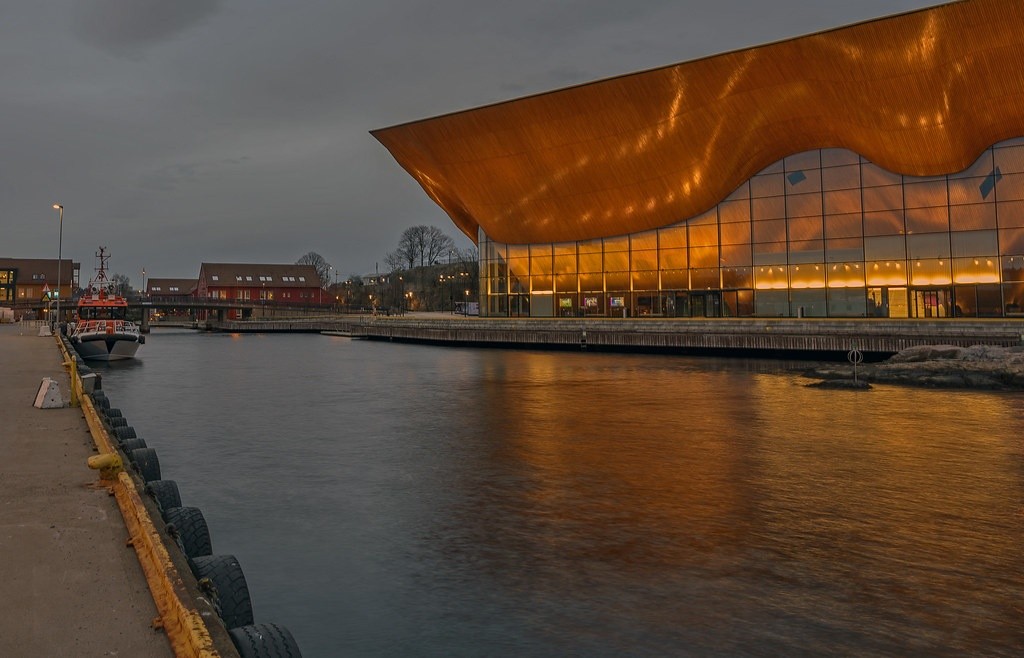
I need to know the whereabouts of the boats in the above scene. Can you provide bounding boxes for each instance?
[67,246,146,363]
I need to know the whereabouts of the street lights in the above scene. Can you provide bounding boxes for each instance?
[52,204,63,336]
[440,275,446,313]
[345,280,351,314]
[448,275,455,315]
[399,276,404,316]
[460,272,468,317]
